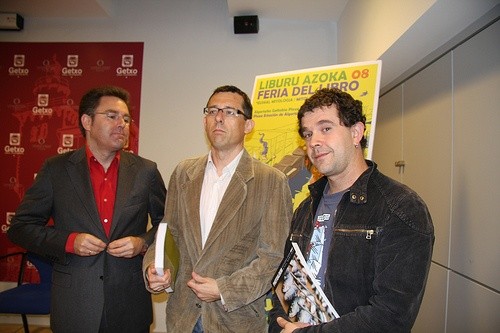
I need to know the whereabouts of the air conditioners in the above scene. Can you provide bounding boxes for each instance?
[0,13,23,30]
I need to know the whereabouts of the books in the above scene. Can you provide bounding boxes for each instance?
[271,240,341,327]
[155,222,181,294]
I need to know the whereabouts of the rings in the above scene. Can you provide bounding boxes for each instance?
[163,285,165,289]
[89,251,92,256]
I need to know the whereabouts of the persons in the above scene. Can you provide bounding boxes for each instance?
[6,86,168,333]
[142,85,294,333]
[267,88,436,332]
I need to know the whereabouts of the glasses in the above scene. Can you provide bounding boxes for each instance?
[204,107,250,119]
[94,112,134,124]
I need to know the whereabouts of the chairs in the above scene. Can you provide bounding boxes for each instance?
[0,252,52,332]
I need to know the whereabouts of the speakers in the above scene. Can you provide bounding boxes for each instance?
[234,15,260,34]
